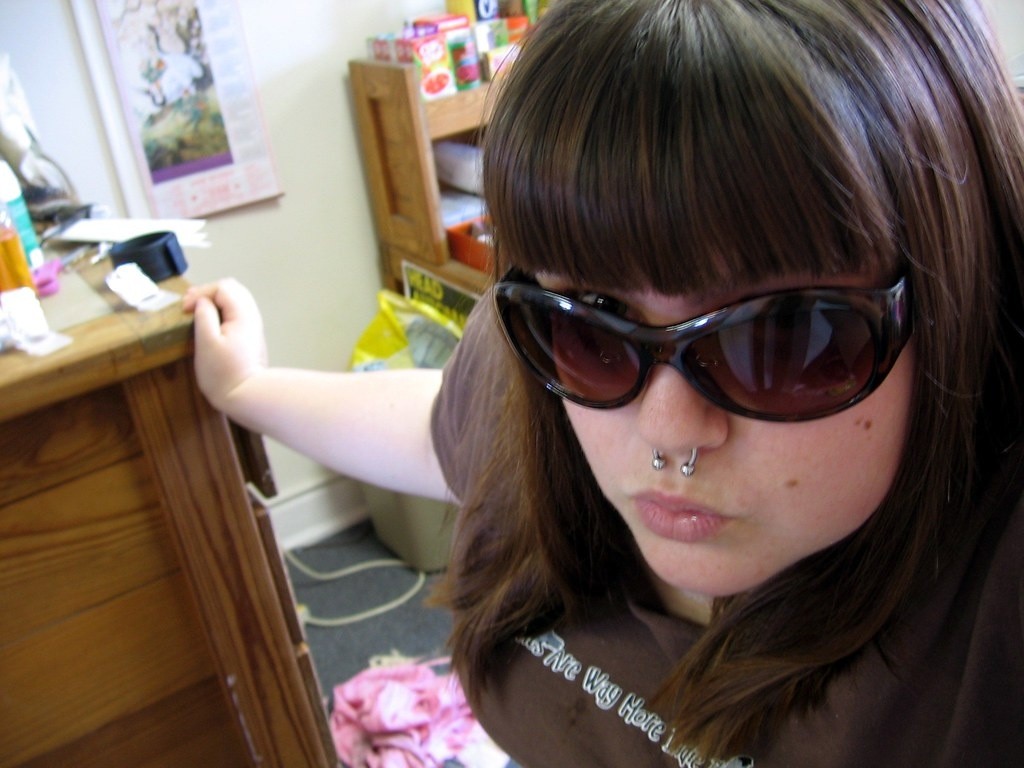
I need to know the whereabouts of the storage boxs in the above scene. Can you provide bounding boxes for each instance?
[366,0,529,107]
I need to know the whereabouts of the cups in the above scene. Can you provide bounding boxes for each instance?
[108,230,188,283]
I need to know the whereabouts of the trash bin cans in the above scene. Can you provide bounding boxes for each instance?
[336,290,464,570]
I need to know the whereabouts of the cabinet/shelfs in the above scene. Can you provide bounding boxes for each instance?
[346,57,506,334]
[0,219,340,768]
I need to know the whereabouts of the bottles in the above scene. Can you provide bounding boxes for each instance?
[0,157,44,302]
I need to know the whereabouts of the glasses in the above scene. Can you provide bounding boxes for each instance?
[492,264,918,422]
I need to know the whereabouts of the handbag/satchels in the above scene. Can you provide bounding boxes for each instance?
[351,291,463,571]
[328,655,477,768]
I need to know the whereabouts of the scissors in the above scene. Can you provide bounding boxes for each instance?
[30,246,85,297]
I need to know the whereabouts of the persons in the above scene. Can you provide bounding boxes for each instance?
[181,0,1024,768]
